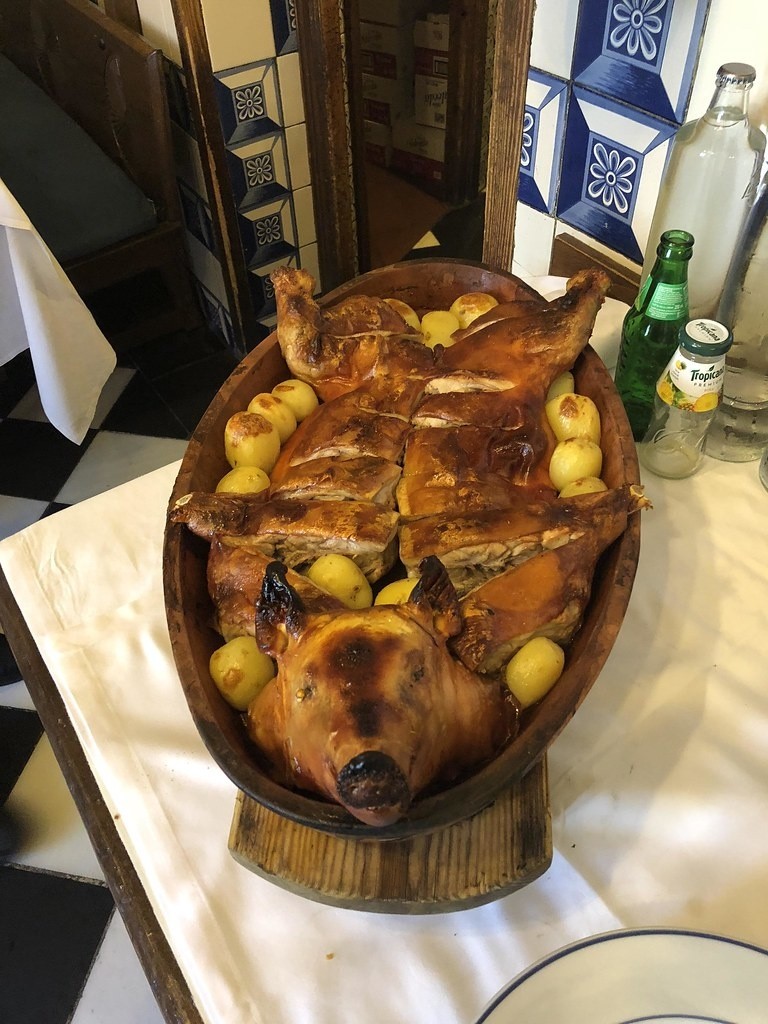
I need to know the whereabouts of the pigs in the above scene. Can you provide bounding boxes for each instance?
[170,266,650,829]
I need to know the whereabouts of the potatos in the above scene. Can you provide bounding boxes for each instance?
[209,291,605,709]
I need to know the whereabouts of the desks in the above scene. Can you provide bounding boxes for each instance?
[0,399,768,1024]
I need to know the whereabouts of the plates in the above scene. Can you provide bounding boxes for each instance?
[466,926,768,1024]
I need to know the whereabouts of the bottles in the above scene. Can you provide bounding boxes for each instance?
[616,229,696,444]
[637,62,768,322]
[695,178,768,462]
[636,319,733,479]
